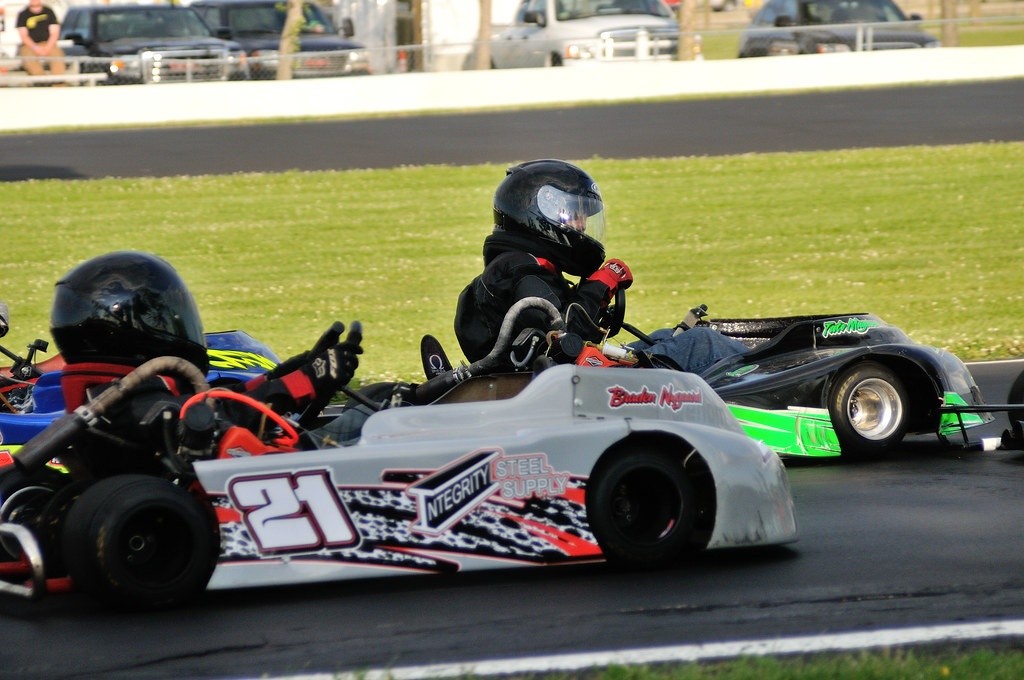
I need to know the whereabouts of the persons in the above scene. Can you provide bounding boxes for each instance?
[49,251,382,461]
[16,0,65,86]
[454,158,752,375]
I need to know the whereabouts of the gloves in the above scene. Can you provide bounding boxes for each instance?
[588,258,633,293]
[311,320,362,387]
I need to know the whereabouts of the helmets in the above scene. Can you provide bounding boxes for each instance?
[52,251,210,375]
[494,158,609,275]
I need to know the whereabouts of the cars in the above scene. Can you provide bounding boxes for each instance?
[737,0,942,63]
[486,0,682,68]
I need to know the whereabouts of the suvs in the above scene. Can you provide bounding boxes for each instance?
[58,5,253,86]
[190,0,374,83]
